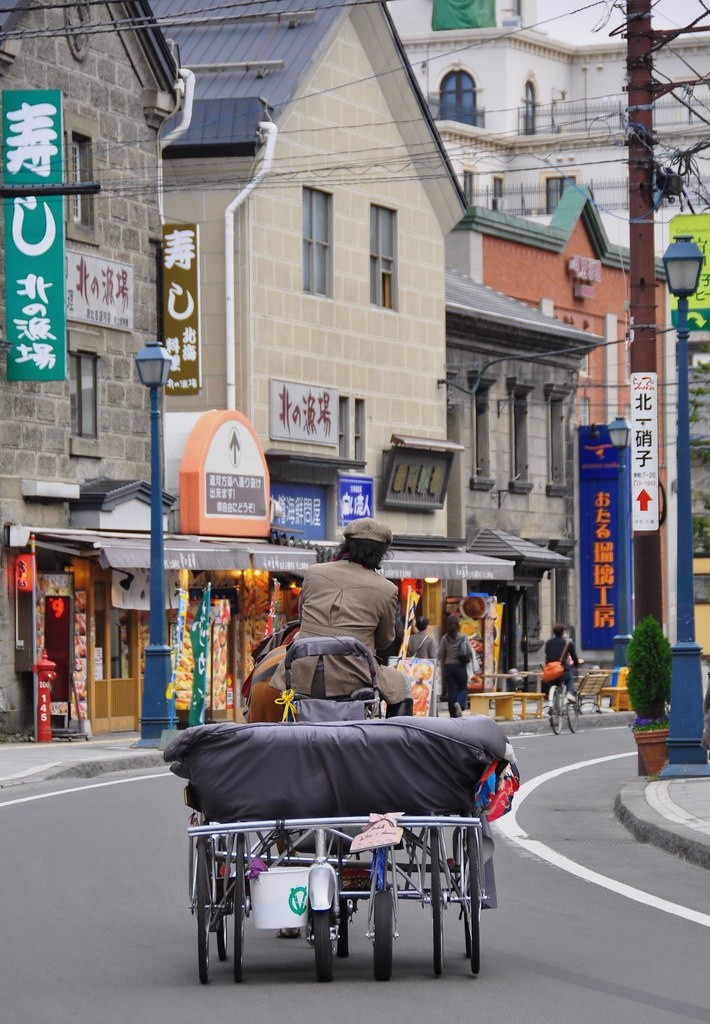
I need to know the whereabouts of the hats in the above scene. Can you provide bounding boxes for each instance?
[343,517,393,550]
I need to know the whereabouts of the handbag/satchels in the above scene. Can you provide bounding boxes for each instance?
[541,660,563,682]
[459,636,470,663]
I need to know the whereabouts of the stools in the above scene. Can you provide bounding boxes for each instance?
[600,686,632,711]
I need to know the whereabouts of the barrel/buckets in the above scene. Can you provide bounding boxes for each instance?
[245,866,309,928]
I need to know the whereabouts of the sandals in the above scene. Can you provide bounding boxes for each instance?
[277,928,300,937]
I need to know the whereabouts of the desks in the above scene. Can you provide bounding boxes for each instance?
[479,672,527,692]
[517,671,544,693]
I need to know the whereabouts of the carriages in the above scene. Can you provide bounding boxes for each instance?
[161,619,522,985]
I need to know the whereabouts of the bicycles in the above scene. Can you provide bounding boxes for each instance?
[548,663,584,735]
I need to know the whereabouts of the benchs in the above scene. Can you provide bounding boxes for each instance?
[466,689,548,721]
[572,673,610,715]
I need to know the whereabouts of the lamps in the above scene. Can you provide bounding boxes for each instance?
[79,557,134,590]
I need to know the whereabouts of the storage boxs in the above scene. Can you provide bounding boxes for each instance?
[624,615,672,777]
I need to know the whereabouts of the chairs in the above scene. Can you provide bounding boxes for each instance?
[280,636,381,724]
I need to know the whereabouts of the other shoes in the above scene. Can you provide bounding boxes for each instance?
[566,693,576,703]
[454,702,462,717]
[550,717,558,727]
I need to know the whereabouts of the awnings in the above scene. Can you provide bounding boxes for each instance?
[9,525,515,581]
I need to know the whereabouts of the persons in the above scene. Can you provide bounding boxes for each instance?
[545,624,578,726]
[267,517,413,719]
[405,616,473,718]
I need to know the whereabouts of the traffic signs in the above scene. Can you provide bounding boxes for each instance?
[630,372,660,531]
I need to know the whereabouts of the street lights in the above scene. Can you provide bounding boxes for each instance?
[131,340,184,749]
[656,233,709,781]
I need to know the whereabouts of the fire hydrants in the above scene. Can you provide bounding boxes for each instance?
[35,648,58,742]
